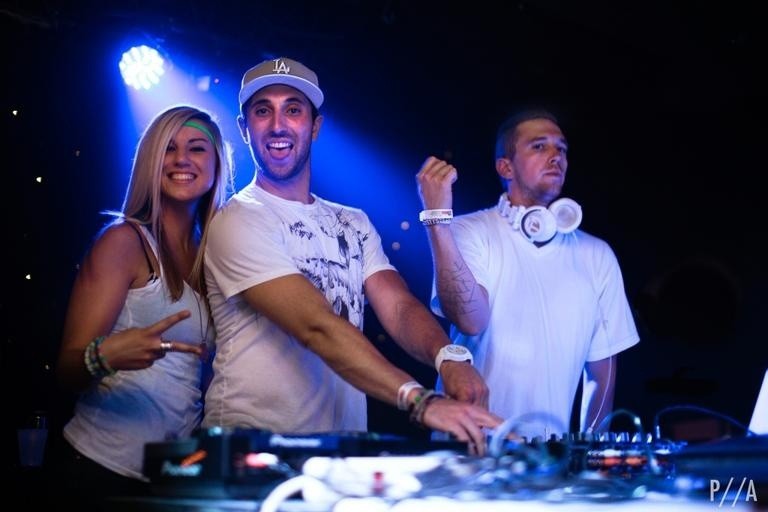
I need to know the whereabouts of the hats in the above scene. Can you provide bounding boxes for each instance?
[237,56,325,112]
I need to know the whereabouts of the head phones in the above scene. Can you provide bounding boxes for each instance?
[497,192,583,246]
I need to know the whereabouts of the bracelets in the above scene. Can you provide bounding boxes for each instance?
[421,218,451,226]
[82,333,117,384]
[396,381,450,426]
[419,208,455,221]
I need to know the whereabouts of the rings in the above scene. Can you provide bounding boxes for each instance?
[161,338,171,352]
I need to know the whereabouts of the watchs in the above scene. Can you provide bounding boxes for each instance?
[433,342,475,375]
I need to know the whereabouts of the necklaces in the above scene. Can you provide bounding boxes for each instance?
[191,286,213,363]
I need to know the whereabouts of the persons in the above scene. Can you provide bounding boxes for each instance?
[414,109,644,442]
[56,104,238,511]
[197,56,521,458]
